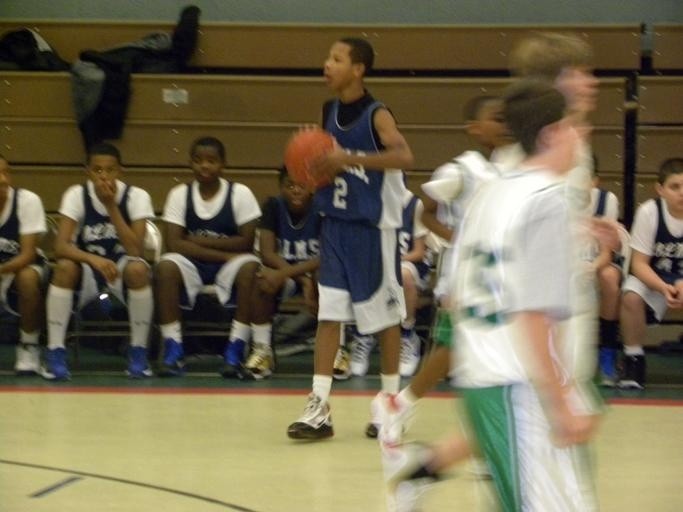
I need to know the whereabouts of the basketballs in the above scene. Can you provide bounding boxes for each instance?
[284,131,334,191]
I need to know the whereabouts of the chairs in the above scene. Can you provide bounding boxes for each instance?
[39,216,442,378]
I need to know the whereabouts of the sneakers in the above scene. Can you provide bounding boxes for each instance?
[349,333,377,376]
[219,337,250,379]
[124,344,153,378]
[596,343,646,388]
[398,329,422,377]
[288,393,334,437]
[240,340,274,379]
[41,346,71,381]
[155,336,184,376]
[14,342,42,374]
[332,346,352,380]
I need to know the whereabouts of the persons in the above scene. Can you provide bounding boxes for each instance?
[0,37,683,512]
